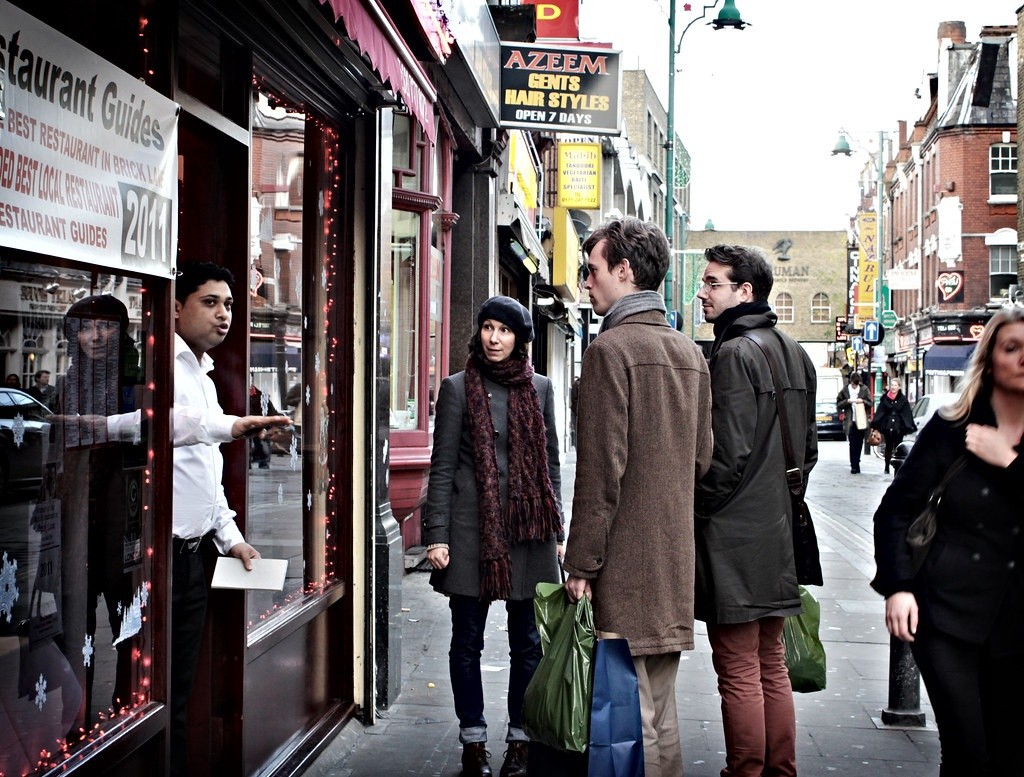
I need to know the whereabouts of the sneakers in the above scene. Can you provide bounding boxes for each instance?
[460,742,492,777]
[497,739,529,777]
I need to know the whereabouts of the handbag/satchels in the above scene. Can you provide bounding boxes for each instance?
[586,628,645,776]
[791,497,825,586]
[525,577,594,753]
[866,429,882,446]
[17,533,62,701]
[901,403,984,569]
[781,586,827,693]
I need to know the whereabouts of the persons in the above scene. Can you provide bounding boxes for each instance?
[424,296,566,777]
[873,378,917,474]
[43,295,146,745]
[691,244,820,777]
[566,218,716,777]
[171,264,294,777]
[871,302,1024,777]
[836,373,873,473]
[4,369,56,413]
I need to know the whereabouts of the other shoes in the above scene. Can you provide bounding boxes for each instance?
[884,460,890,474]
[851,464,860,474]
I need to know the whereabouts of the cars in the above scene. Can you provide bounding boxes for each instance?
[815,400,845,439]
[892,394,967,474]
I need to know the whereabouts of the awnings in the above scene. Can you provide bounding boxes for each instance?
[924,341,976,376]
[206,0,446,149]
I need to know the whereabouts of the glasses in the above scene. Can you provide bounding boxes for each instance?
[696,280,739,293]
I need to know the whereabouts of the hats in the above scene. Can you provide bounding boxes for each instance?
[480,295,538,338]
[62,295,129,341]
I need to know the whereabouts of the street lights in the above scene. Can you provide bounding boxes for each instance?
[831,125,886,421]
[663,0,753,321]
